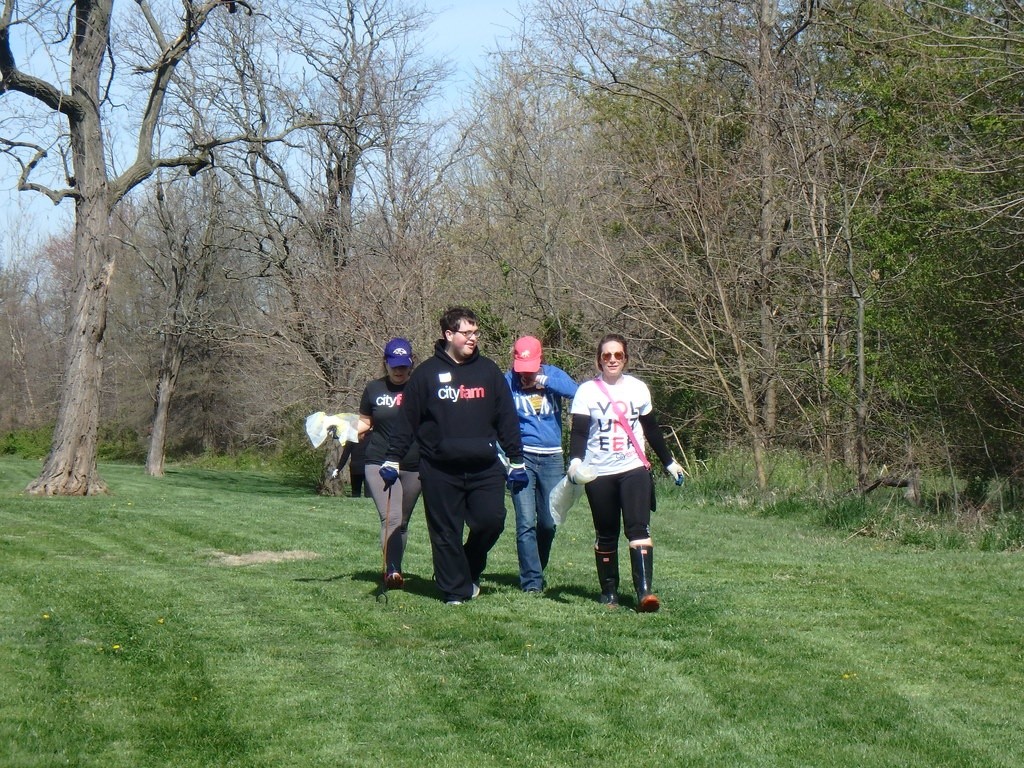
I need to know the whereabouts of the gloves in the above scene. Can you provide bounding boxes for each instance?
[327,425,340,440]
[567,457,582,485]
[506,463,529,495]
[379,461,399,492]
[665,460,684,486]
[332,469,338,479]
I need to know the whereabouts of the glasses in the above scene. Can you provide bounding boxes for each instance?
[602,351,625,361]
[456,329,481,339]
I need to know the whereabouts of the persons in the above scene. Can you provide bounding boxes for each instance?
[496,335,578,594]
[379,306,529,606]
[327,338,421,587]
[568,334,687,612]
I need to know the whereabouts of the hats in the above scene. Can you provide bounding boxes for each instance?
[513,335,542,373]
[385,338,413,369]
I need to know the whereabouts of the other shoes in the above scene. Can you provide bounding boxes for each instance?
[472,578,481,599]
[386,571,403,589]
[446,601,463,606]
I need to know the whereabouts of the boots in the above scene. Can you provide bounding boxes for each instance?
[595,548,619,609]
[629,544,660,612]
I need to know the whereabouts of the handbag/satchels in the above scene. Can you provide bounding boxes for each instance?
[643,462,656,512]
